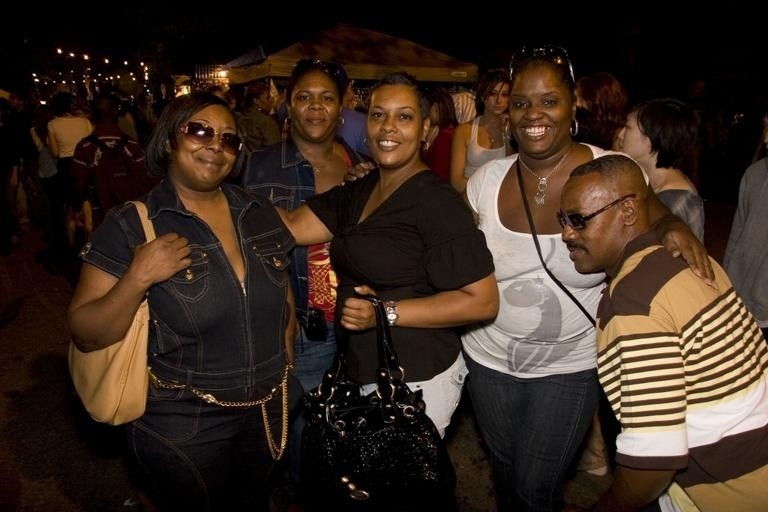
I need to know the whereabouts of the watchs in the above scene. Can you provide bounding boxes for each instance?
[386,299,398,327]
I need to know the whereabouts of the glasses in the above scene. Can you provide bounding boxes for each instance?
[502,42,579,90]
[173,121,245,155]
[556,192,638,231]
[288,57,348,107]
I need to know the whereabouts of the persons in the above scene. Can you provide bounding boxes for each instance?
[136,92,156,125]
[222,57,376,459]
[558,154,767,512]
[274,71,500,511]
[114,95,138,142]
[419,86,457,184]
[65,90,298,511]
[234,80,280,153]
[719,112,767,343]
[6,89,51,228]
[618,98,706,247]
[71,91,157,242]
[334,67,370,168]
[47,90,93,249]
[574,67,628,151]
[462,45,718,511]
[449,67,516,201]
[0,96,15,253]
[207,86,235,111]
[2,79,128,107]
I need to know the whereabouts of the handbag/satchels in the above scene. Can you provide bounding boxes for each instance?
[68,200,160,426]
[302,289,457,512]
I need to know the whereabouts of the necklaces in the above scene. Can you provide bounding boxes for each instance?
[483,117,501,145]
[302,142,335,175]
[516,149,572,208]
